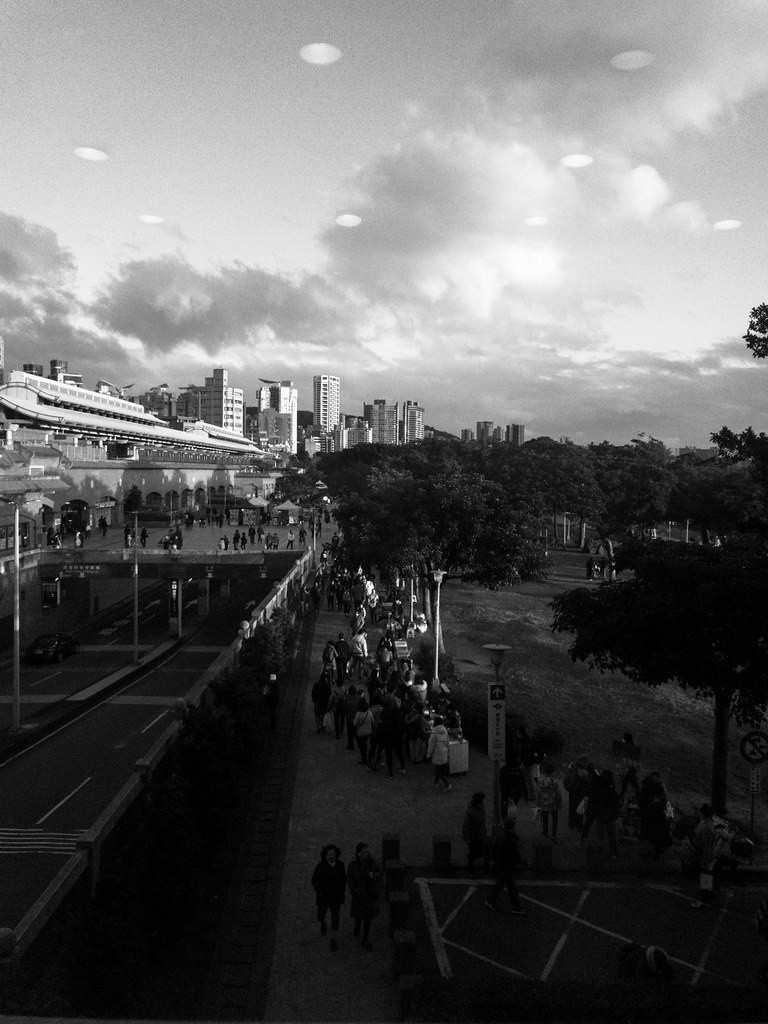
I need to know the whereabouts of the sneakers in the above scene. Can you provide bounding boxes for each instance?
[361,939,373,949]
[319,920,328,935]
[328,937,339,952]
[317,725,321,733]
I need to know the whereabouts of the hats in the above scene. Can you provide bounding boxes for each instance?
[698,801,715,819]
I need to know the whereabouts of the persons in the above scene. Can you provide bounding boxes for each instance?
[0,473,768,1023]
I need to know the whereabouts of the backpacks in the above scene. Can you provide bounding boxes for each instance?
[334,689,347,712]
[323,648,334,661]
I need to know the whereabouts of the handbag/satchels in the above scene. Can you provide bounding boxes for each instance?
[576,797,589,815]
[508,800,519,819]
[531,807,543,823]
[697,872,714,891]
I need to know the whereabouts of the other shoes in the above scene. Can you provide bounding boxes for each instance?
[429,782,440,788]
[400,767,407,775]
[484,899,498,908]
[366,766,376,773]
[441,783,452,792]
[385,773,393,779]
[511,907,529,913]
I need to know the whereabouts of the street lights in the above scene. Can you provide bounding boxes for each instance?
[430,568,448,693]
[482,641,513,831]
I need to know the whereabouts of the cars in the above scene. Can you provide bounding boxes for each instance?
[26,632,79,663]
